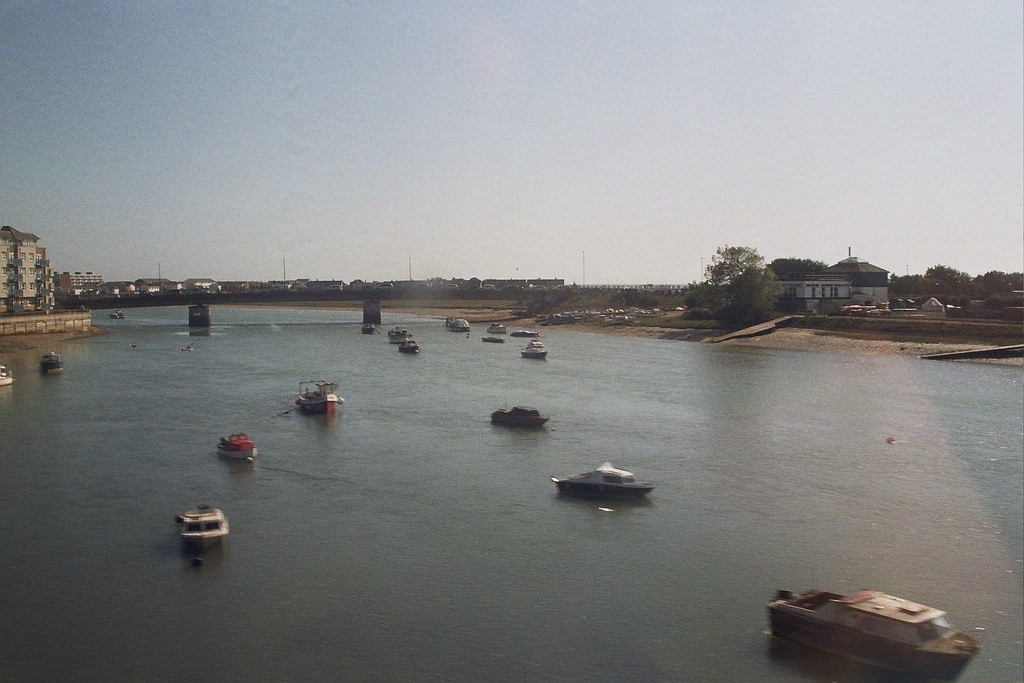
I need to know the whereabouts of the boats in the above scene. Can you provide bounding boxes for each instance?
[129,343,137,349]
[40,352,65,373]
[181,347,193,352]
[294,379,339,414]
[388,326,413,344]
[450,319,471,333]
[766,586,981,683]
[489,406,548,427]
[550,467,654,499]
[521,340,547,360]
[361,322,376,335]
[108,310,125,320]
[481,323,541,343]
[0,365,15,386]
[605,315,636,324]
[399,338,419,353]
[445,317,455,327]
[217,433,256,461]
[173,503,230,550]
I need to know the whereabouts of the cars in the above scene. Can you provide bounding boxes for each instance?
[324,282,569,290]
